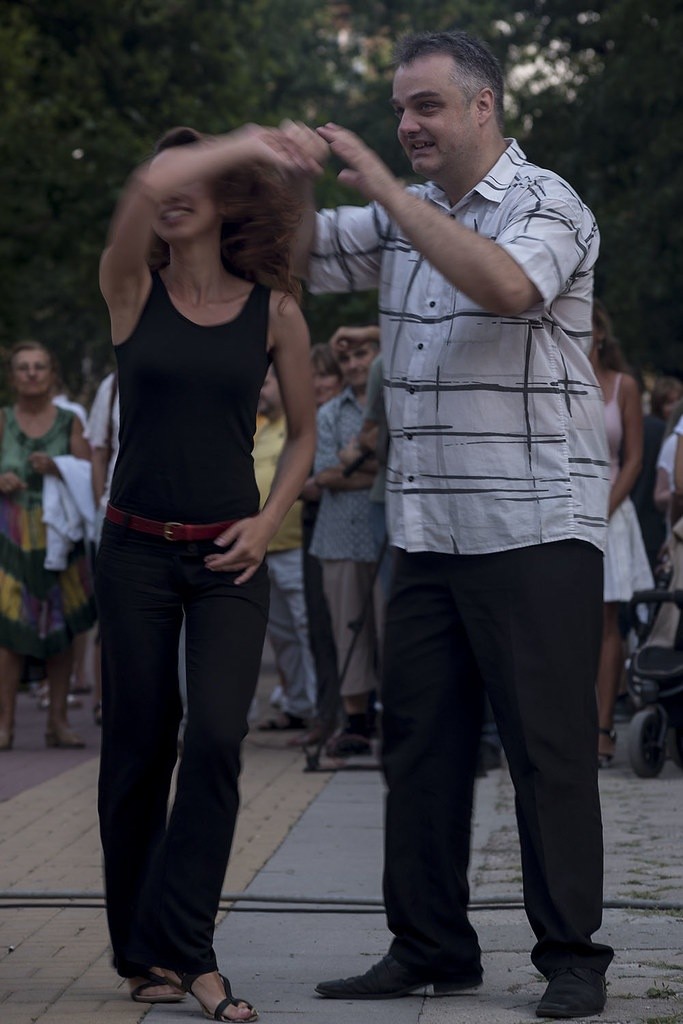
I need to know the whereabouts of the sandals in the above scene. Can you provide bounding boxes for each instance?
[155,968,259,1024]
[595,726,617,765]
[129,974,186,1000]
[260,713,310,730]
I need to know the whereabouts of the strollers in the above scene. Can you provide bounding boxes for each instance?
[625,514,683,779]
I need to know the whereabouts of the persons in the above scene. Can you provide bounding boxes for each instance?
[269,26,621,1018]
[0,293,682,779]
[89,116,316,1024]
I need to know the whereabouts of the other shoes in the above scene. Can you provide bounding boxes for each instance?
[30,678,104,725]
[326,734,372,756]
[1,730,13,749]
[46,726,86,749]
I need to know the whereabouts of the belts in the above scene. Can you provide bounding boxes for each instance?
[105,508,239,540]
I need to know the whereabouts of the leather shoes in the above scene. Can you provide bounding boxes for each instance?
[315,953,484,999]
[536,973,606,1016]
